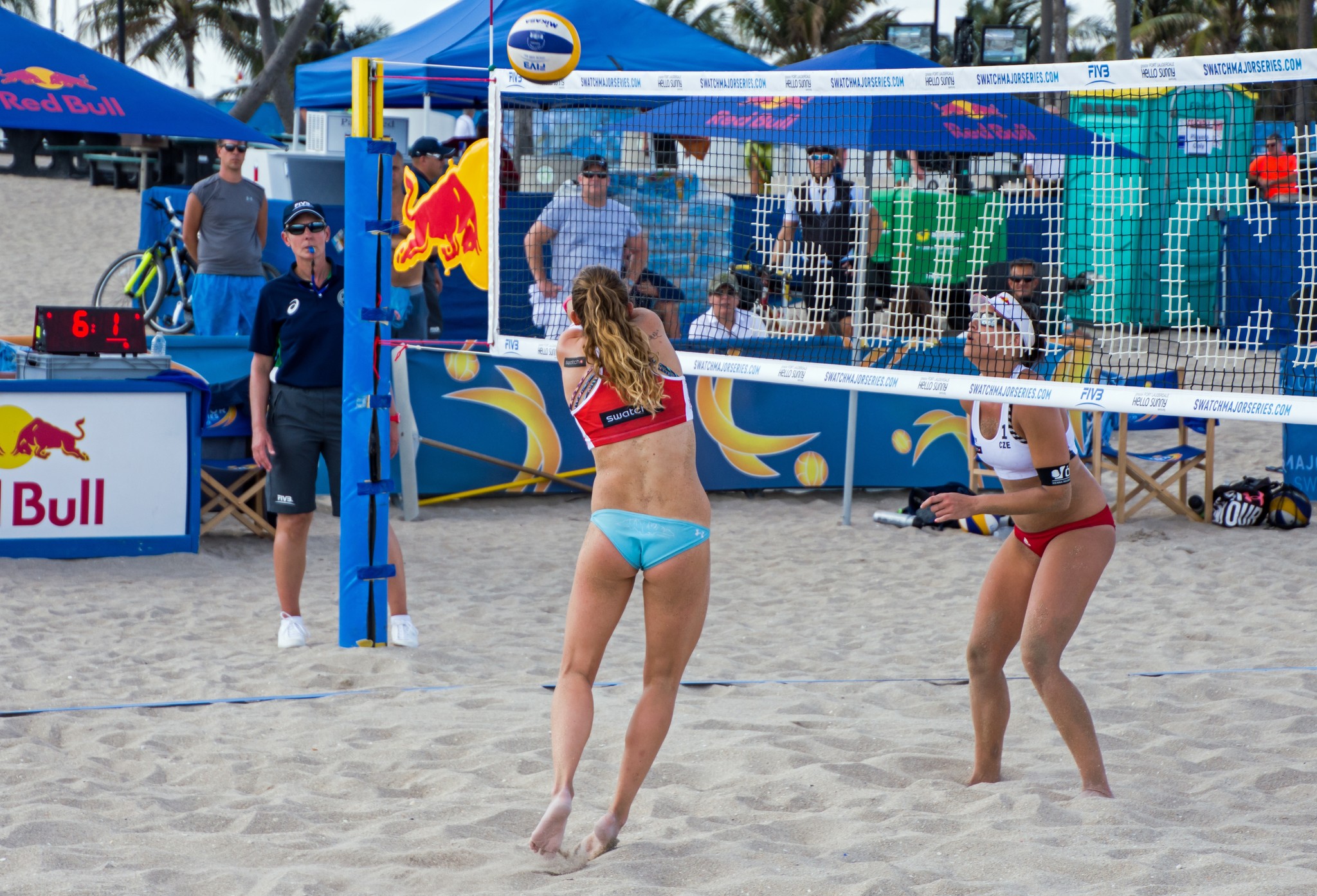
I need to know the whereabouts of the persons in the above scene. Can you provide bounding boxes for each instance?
[183,137,267,337]
[1248,133,1300,204]
[528,265,713,866]
[249,199,419,647]
[387,107,1073,342]
[921,291,1116,797]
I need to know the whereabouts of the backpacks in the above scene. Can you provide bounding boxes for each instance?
[906,481,980,516]
[1208,474,1281,526]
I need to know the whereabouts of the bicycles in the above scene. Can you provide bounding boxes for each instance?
[92,195,281,335]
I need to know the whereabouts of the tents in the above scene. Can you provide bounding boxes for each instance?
[293,0,775,177]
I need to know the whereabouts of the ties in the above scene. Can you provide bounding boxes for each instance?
[818,188,826,218]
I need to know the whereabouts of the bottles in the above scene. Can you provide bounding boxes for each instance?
[993,526,1014,540]
[1188,495,1205,519]
[999,516,1015,526]
[1061,314,1073,334]
[150,331,166,356]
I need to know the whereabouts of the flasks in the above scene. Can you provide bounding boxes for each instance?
[873,510,926,530]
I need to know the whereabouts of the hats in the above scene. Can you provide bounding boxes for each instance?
[969,291,1036,352]
[709,273,739,293]
[583,154,608,169]
[282,199,325,228]
[409,136,455,157]
[805,145,839,156]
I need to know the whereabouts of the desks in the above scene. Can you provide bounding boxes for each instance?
[161,130,281,160]
[0,363,207,564]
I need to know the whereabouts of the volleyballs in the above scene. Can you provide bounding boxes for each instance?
[506,8,581,85]
[1268,490,1312,528]
[957,514,1000,536]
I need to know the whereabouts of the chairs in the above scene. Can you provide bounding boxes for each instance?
[1085,365,1224,526]
[190,374,276,538]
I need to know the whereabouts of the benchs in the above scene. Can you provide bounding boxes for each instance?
[0,136,166,191]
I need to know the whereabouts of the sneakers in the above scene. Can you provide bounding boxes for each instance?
[391,614,420,646]
[278,611,310,649]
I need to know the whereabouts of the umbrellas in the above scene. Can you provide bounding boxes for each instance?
[0,6,290,151]
[595,39,1155,524]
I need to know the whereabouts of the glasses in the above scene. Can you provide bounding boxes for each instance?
[219,143,247,153]
[715,287,737,297]
[1010,275,1034,283]
[583,170,608,179]
[972,311,1018,332]
[807,154,834,162]
[427,152,444,161]
[284,221,326,235]
